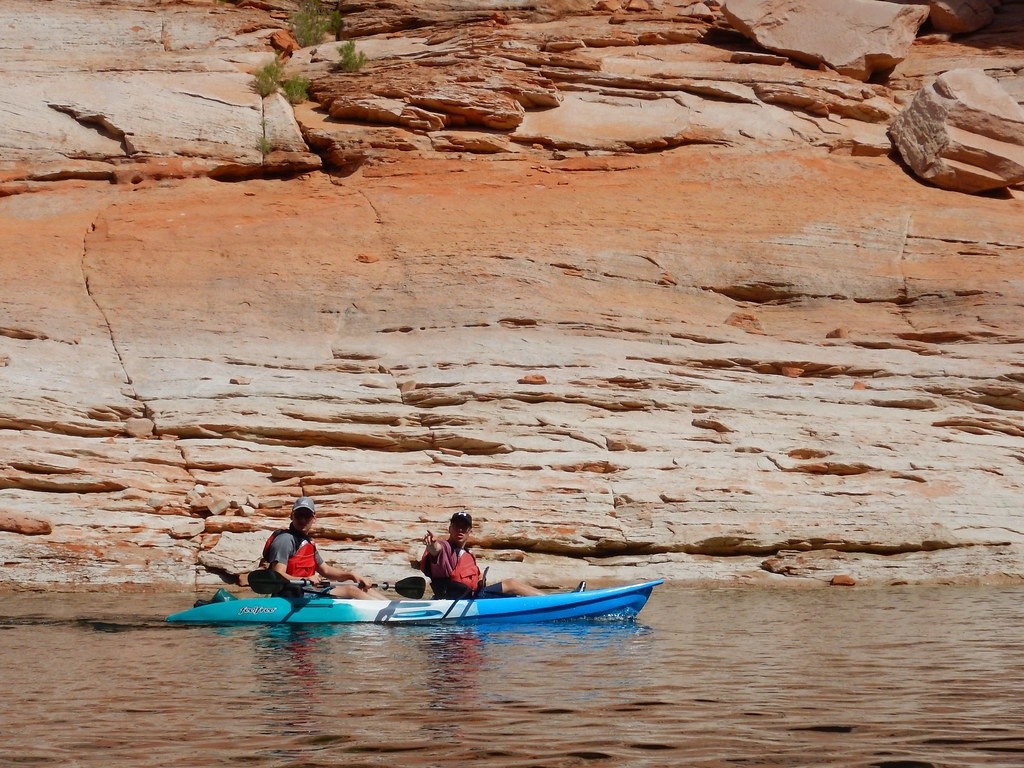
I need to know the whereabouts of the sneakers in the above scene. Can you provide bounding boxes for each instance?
[574,581,586,592]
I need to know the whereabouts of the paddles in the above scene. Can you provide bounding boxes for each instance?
[247,569,426,600]
[477,565,490,598]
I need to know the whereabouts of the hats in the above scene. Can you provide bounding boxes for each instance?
[451,512,472,528]
[293,497,315,512]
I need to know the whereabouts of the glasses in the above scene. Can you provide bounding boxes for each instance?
[293,512,314,519]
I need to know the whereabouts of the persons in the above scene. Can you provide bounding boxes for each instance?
[419,512,587,599]
[262,497,389,601]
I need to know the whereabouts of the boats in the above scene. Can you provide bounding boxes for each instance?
[163,579,667,627]
